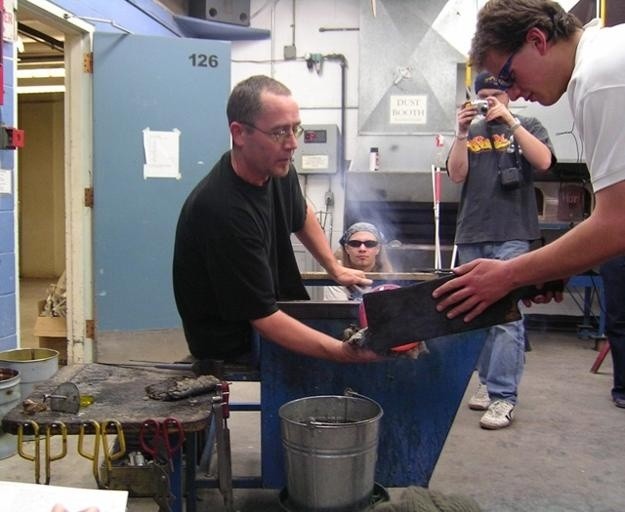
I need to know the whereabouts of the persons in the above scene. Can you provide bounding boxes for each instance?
[446,72,557,430]
[323,222,390,302]
[599,260,624,408]
[172,75,392,368]
[432,0,625,323]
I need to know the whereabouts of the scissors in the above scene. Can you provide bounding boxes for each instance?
[18,417,185,512]
[216,381,234,512]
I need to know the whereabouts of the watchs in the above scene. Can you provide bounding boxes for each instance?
[511,117,521,133]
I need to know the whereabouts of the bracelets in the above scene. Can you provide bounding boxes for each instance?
[456,135,469,141]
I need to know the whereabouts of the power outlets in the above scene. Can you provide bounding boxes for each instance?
[325,192,334,205]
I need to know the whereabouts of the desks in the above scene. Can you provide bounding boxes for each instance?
[0,365,233,512]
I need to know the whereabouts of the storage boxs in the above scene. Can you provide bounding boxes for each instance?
[33,299,67,364]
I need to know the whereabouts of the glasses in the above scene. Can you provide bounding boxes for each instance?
[347,239,380,248]
[240,120,306,142]
[497,48,518,92]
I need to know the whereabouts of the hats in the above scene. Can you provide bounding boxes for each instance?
[475,72,505,95]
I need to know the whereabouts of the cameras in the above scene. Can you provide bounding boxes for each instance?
[470,99,490,117]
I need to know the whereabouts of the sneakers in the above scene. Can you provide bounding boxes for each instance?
[467,383,492,410]
[612,387,625,409]
[479,401,514,430]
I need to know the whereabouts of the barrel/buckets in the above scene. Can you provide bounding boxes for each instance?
[0,347,61,442]
[277,389,385,510]
[0,366,22,462]
